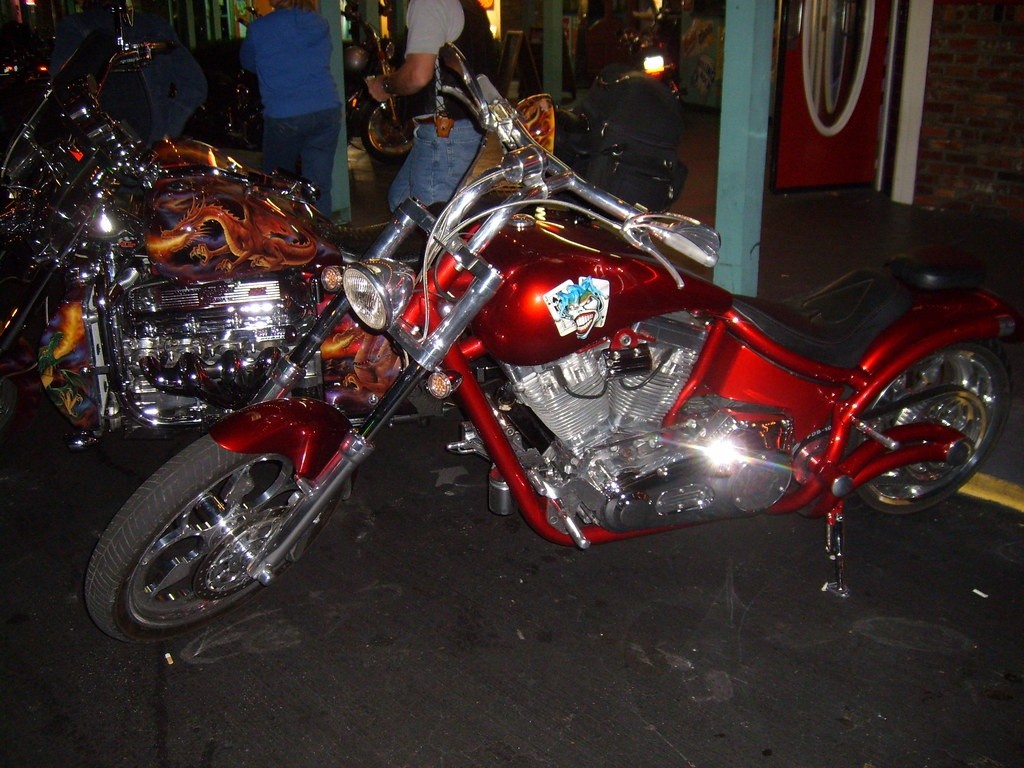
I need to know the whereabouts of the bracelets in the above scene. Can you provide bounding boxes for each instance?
[383,76,393,93]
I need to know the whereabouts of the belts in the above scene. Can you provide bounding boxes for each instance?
[415,115,462,124]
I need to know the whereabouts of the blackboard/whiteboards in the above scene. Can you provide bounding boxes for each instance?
[492,30,542,99]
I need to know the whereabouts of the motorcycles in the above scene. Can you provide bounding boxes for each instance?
[0,0,691,454]
[81,37,1023,647]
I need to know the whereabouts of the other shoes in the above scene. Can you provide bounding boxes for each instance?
[392,379,444,417]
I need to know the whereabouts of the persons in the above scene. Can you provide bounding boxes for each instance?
[239,0,342,217]
[32,0,208,144]
[367,0,495,214]
